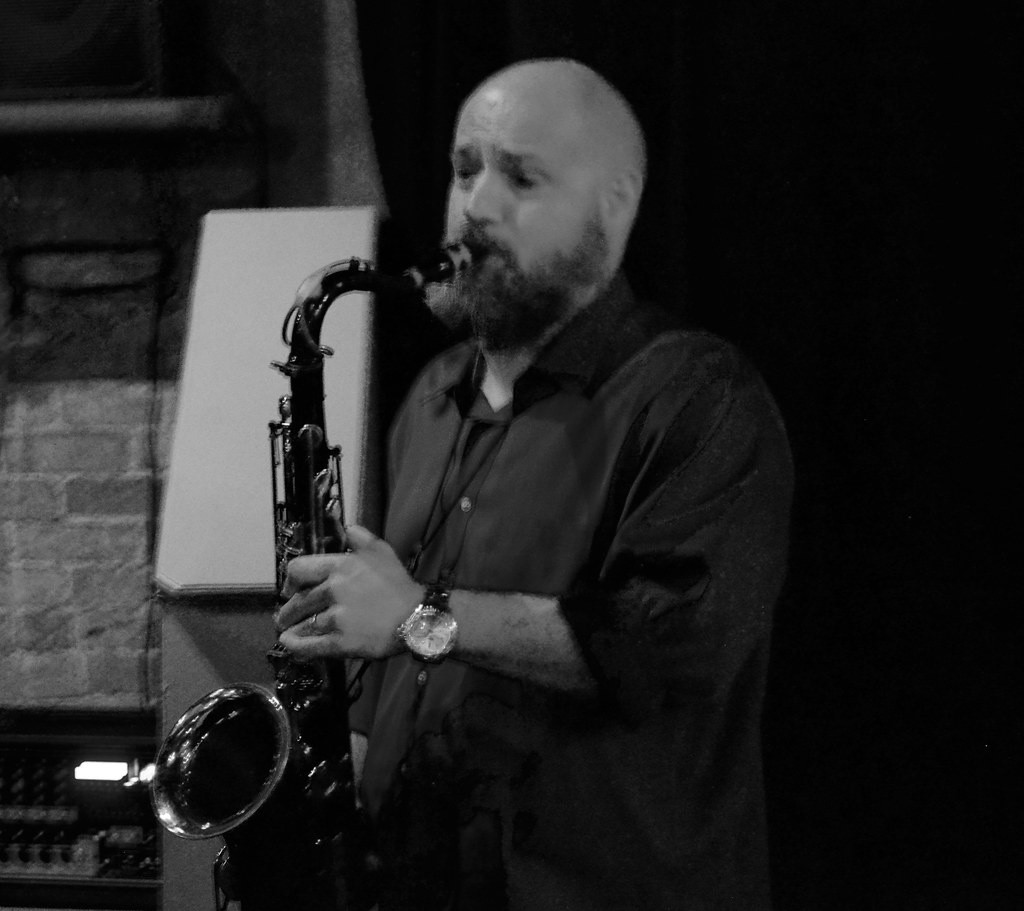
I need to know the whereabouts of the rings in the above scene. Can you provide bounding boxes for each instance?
[310,612,325,635]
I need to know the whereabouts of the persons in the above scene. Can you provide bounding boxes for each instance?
[274,56,792,910]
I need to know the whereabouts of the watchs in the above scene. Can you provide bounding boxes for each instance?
[400,584,463,668]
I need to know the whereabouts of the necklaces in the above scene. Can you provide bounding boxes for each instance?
[404,339,507,576]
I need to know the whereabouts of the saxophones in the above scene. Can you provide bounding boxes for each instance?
[151,237,487,911]
